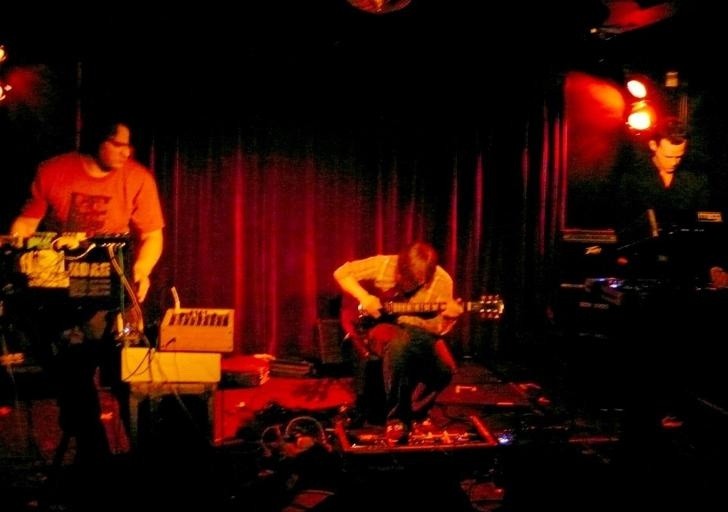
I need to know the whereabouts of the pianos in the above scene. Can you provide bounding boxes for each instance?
[616,229,728,293]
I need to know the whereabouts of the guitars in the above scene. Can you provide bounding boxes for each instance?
[342,279,505,327]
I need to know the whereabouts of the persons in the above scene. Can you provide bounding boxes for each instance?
[1,123,168,457]
[611,115,728,287]
[332,241,457,446]
